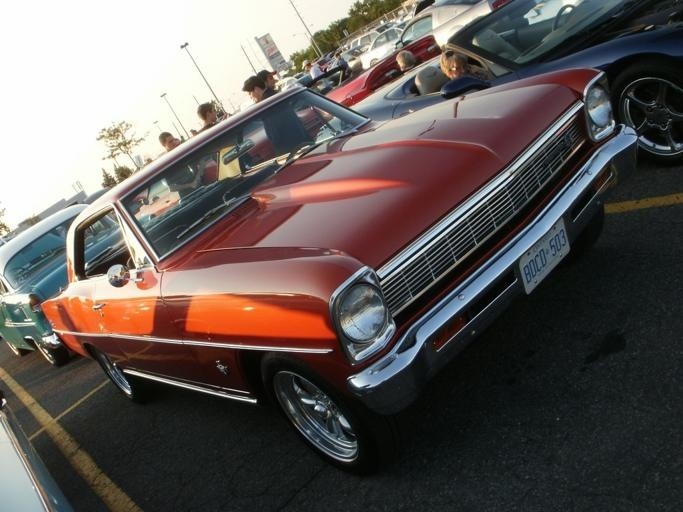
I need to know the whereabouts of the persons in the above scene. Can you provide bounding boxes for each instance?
[241,75,315,156]
[157,132,209,200]
[254,69,277,92]
[195,103,233,132]
[334,52,356,79]
[300,59,333,95]
[439,48,491,81]
[392,50,421,75]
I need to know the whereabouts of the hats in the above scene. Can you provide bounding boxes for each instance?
[256,69,277,81]
[302,60,311,70]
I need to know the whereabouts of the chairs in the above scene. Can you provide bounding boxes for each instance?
[472,27,520,77]
[414,65,450,95]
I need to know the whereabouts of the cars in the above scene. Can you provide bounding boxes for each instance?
[0,0,679,483]
